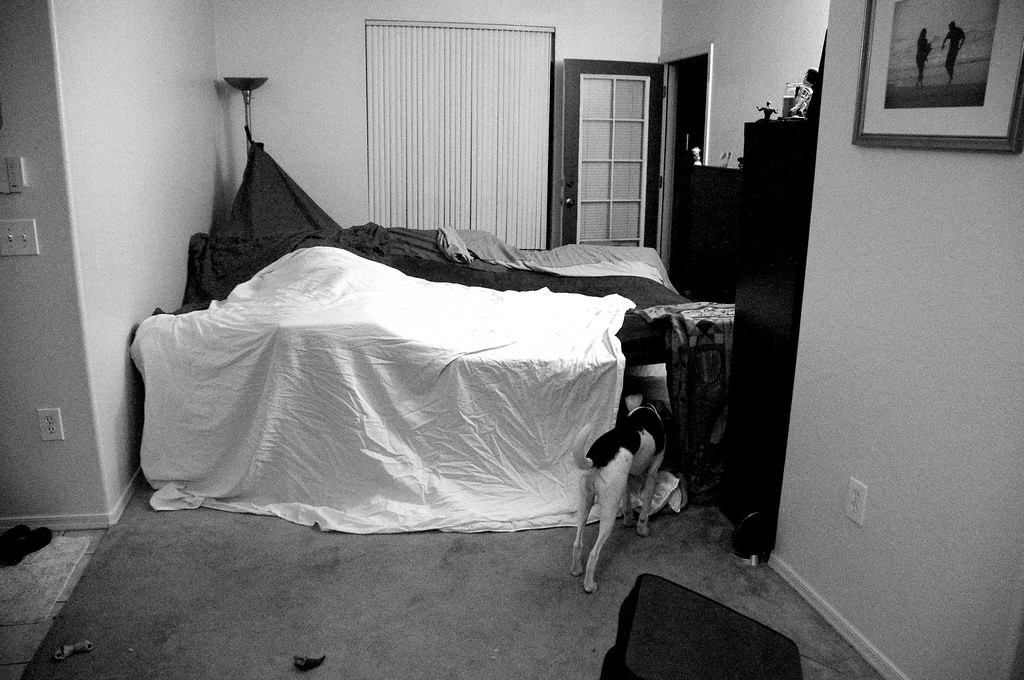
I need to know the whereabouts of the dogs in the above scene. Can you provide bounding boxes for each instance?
[570,398,667,593]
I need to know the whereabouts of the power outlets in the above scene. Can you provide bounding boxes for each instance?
[38,407,65,442]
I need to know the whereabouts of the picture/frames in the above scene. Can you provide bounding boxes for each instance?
[845,3,1024,159]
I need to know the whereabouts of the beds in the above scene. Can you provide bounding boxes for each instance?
[136,224,702,503]
